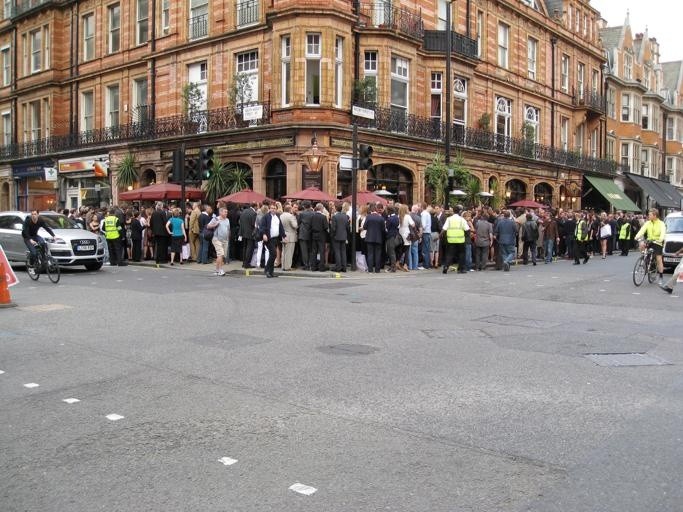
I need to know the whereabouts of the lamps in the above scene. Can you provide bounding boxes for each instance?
[297,130,329,174]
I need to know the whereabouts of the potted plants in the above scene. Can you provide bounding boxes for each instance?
[179,81,206,135]
[224,71,256,129]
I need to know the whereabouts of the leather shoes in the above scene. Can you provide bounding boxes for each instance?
[264,270,277,278]
[658,284,672,293]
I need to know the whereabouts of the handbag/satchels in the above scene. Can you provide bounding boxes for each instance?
[394,233,404,250]
[252,228,269,241]
[600,224,612,239]
[409,232,418,241]
[182,243,191,259]
[204,225,213,240]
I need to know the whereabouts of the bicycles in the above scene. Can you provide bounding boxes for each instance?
[630,238,659,285]
[22,238,62,285]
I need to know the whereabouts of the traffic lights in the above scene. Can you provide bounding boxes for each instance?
[196,148,214,182]
[357,144,374,172]
[188,156,200,180]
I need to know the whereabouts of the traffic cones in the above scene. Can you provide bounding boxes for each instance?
[0,263,17,308]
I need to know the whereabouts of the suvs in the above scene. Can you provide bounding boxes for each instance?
[0,209,104,273]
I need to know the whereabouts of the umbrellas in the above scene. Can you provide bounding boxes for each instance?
[508,198,549,208]
[119,180,207,200]
[342,188,390,207]
[280,184,341,201]
[215,188,276,204]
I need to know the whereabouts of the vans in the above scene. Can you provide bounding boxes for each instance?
[658,211,682,269]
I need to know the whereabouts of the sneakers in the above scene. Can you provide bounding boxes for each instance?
[28,267,36,276]
[215,270,225,276]
[443,267,447,273]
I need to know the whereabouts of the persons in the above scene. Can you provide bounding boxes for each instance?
[22,209,56,275]
[634,208,666,285]
[658,247,683,293]
